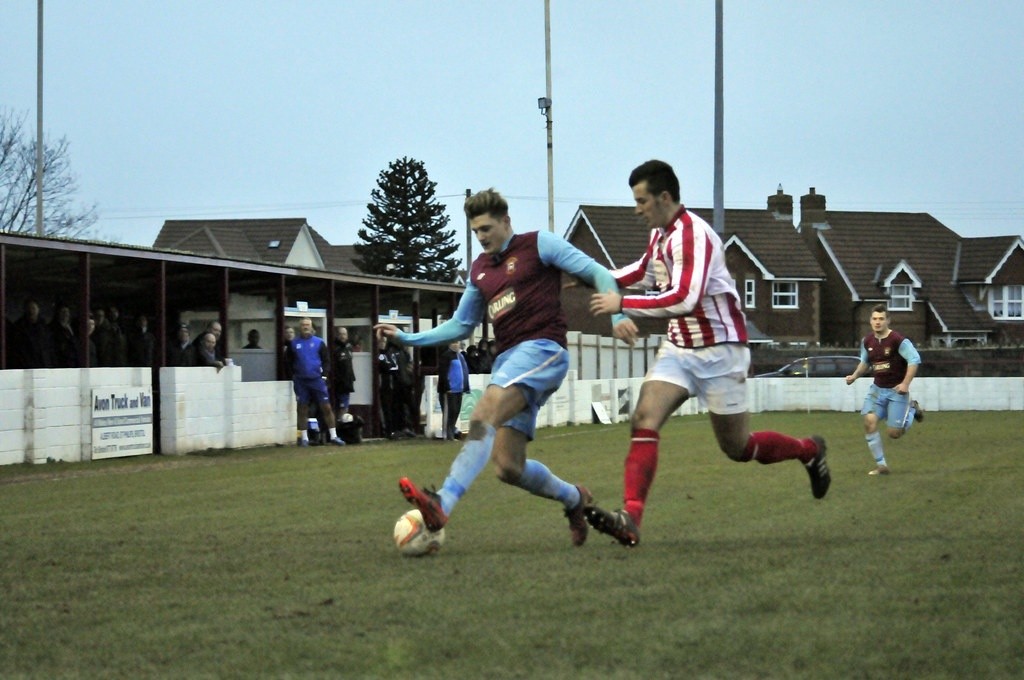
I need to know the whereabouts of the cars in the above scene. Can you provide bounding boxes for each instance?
[755,356,872,378]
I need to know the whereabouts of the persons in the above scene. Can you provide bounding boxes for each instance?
[191,320,223,365]
[281,325,298,380]
[5,297,162,385]
[349,334,364,352]
[461,339,497,374]
[329,326,355,420]
[165,327,197,366]
[195,333,227,368]
[437,339,471,441]
[562,159,831,548]
[374,187,639,547]
[242,329,263,349]
[375,335,417,440]
[290,319,345,447]
[846,304,924,475]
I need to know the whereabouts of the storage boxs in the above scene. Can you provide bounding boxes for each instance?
[306,418,319,431]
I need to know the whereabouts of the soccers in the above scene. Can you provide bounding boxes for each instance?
[393,508,446,556]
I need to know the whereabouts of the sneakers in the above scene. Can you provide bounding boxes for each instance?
[910,401,924,424]
[566,485,592,546]
[868,465,889,474]
[400,477,450,532]
[331,438,344,445]
[301,439,309,446]
[582,509,640,546]
[804,434,831,500]
